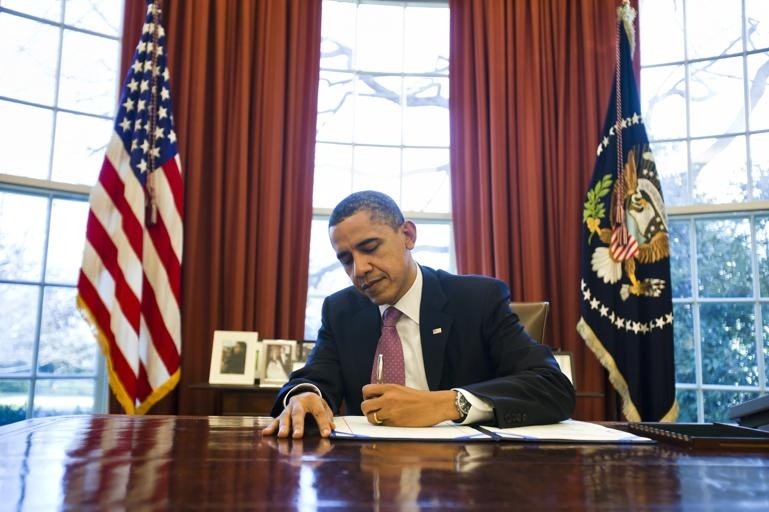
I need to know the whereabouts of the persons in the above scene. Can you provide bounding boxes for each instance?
[260,191,577,440]
[266,345,289,381]
[221,342,247,374]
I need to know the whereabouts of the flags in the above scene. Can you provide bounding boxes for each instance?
[75,0,184,415]
[577,0,679,422]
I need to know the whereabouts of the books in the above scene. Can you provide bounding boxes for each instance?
[328,415,654,442]
[628,421,769,450]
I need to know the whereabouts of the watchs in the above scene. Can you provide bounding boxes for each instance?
[452,389,472,423]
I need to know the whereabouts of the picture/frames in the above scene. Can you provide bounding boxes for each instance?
[208,330,254,386]
[300,339,318,362]
[259,340,298,387]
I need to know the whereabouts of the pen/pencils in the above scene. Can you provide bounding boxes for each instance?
[375,354,383,384]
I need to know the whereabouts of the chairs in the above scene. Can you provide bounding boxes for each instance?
[511,300,549,341]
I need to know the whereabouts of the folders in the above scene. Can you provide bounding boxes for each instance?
[325,416,660,447]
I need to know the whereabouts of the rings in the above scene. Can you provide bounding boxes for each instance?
[373,411,383,424]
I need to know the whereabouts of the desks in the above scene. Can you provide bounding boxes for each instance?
[0,414,769,512]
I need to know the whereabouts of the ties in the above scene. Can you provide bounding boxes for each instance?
[370,306,406,387]
